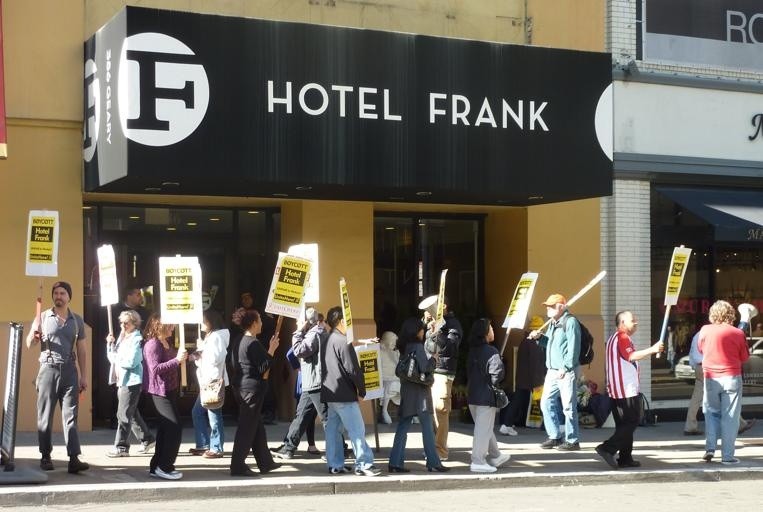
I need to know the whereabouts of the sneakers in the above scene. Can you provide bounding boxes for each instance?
[269,446,293,460]
[202,449,224,458]
[106,445,129,457]
[541,438,562,449]
[486,453,512,468]
[354,465,382,477]
[137,440,156,453]
[703,449,715,462]
[330,465,353,475]
[558,440,581,452]
[189,447,209,455]
[721,457,740,466]
[499,424,518,436]
[470,461,498,473]
[150,465,184,480]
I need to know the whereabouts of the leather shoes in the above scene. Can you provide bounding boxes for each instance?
[67,459,89,474]
[595,443,618,470]
[738,417,756,436]
[40,458,55,470]
[307,446,321,455]
[618,459,641,469]
[232,468,257,476]
[341,446,354,454]
[684,430,704,435]
[259,461,283,474]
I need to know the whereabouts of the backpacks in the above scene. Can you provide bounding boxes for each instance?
[563,313,594,365]
[636,391,650,426]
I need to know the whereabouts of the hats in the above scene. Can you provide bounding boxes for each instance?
[528,315,546,331]
[542,292,566,307]
[51,281,72,300]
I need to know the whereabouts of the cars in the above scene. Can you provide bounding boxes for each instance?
[674,334,763,386]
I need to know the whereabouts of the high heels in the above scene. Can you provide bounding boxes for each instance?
[387,463,411,474]
[427,463,450,472]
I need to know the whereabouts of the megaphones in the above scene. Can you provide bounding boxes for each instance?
[737,303,758,331]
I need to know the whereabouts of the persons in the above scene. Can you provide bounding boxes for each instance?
[752,323,763,336]
[25,281,88,474]
[662,320,697,375]
[376,330,427,426]
[527,293,582,450]
[499,316,546,436]
[682,329,755,436]
[420,294,462,462]
[463,317,511,473]
[596,309,666,469]
[385,317,449,472]
[697,297,744,467]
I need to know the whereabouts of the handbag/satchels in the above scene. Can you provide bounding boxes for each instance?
[491,384,509,408]
[198,377,226,411]
[394,354,435,387]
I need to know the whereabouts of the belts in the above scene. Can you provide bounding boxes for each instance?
[696,362,702,366]
